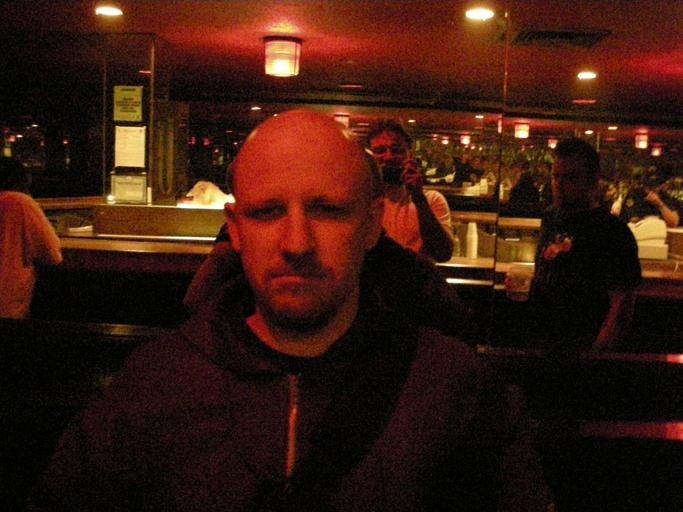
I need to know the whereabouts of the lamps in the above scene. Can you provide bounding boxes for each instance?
[263,36,303,78]
[334,112,349,129]
[514,122,530,139]
[634,133,649,149]
[651,145,663,157]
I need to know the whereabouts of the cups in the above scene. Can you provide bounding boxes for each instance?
[505,262,536,301]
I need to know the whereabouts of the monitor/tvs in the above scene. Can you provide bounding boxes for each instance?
[626,215,669,244]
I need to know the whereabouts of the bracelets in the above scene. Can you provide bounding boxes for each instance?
[658,200,664,209]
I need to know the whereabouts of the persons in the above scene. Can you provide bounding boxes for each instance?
[419,146,614,217]
[26,108,563,510]
[182,162,242,308]
[363,151,477,349]
[612,164,680,228]
[0,155,63,322]
[528,139,642,511]
[366,119,455,263]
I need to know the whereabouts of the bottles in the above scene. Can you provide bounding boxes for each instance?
[467,222,477,260]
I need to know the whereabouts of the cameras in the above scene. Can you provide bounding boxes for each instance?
[382,164,404,182]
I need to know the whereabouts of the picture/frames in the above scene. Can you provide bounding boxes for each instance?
[111,82,149,204]
